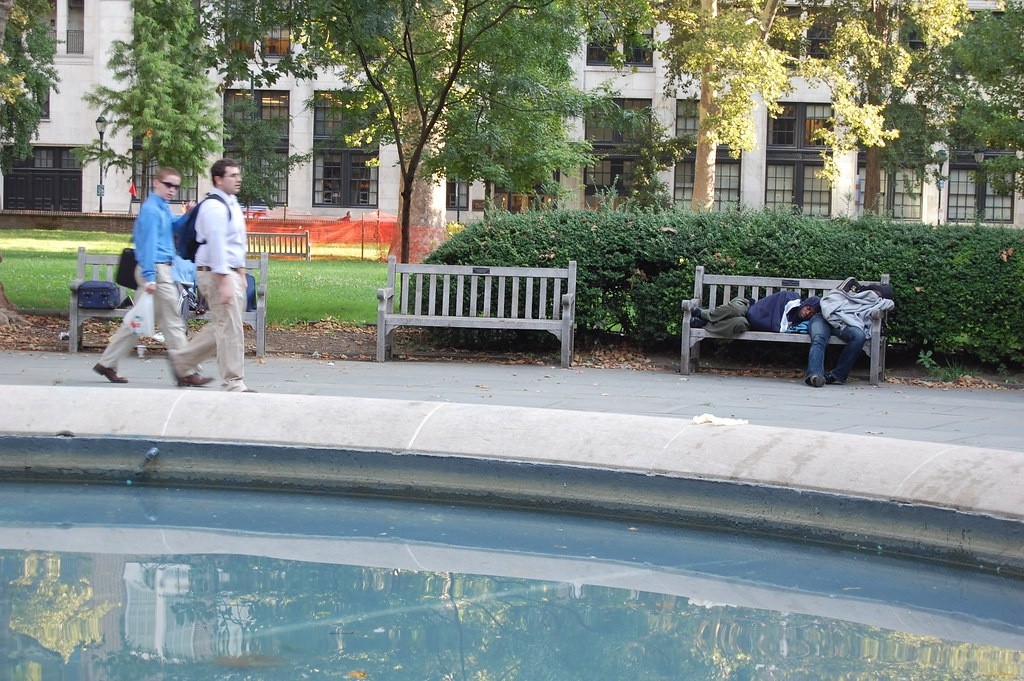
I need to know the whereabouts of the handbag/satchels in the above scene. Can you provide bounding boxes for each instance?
[120,290,156,337]
[115,248,138,290]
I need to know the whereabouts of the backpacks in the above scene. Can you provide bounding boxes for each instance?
[170,194,231,263]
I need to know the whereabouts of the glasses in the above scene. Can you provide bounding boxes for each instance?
[159,179,180,190]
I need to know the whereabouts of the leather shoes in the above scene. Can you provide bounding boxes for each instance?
[178,372,215,387]
[92,363,128,383]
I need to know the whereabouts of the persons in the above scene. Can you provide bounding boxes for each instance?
[805,276,895,388]
[337,211,352,221]
[93,167,216,387]
[166,158,259,394]
[690,290,821,338]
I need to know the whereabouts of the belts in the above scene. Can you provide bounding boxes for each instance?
[197,265,237,271]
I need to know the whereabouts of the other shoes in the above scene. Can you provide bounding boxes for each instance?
[689,307,706,328]
[809,376,826,387]
[825,374,835,384]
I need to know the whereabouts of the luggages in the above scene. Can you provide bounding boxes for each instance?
[77,281,121,309]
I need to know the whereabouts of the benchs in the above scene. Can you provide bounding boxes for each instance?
[376,254,577,368]
[245,230,312,262]
[680,266,891,386]
[70,247,269,357]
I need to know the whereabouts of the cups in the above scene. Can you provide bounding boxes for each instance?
[136,345,147,357]
